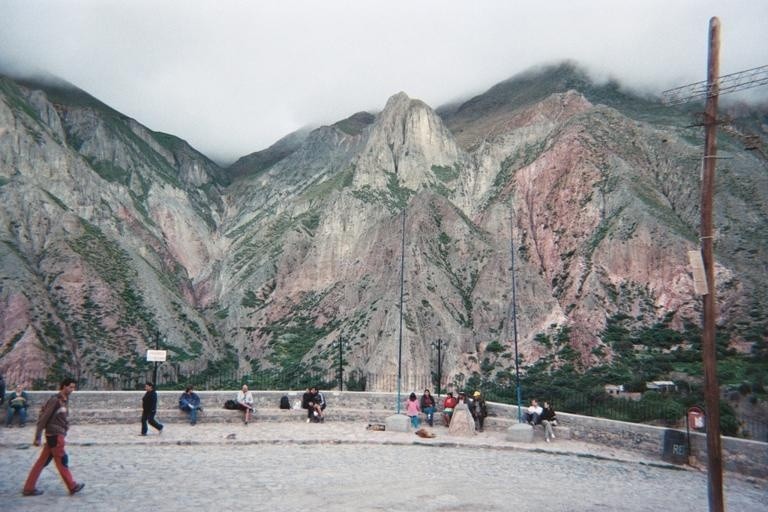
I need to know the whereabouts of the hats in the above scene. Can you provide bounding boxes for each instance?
[472,390,481,396]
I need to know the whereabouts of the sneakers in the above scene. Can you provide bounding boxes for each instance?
[22,487,45,496]
[306,411,325,424]
[545,433,556,443]
[69,482,88,495]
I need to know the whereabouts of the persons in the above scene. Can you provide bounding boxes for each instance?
[301,387,315,423]
[236,384,256,424]
[137,382,163,436]
[178,385,205,425]
[5,383,29,428]
[21,377,85,496]
[540,399,556,442]
[312,386,326,423]
[522,398,544,426]
[404,389,488,432]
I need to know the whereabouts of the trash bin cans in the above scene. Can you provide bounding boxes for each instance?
[662,430,687,464]
